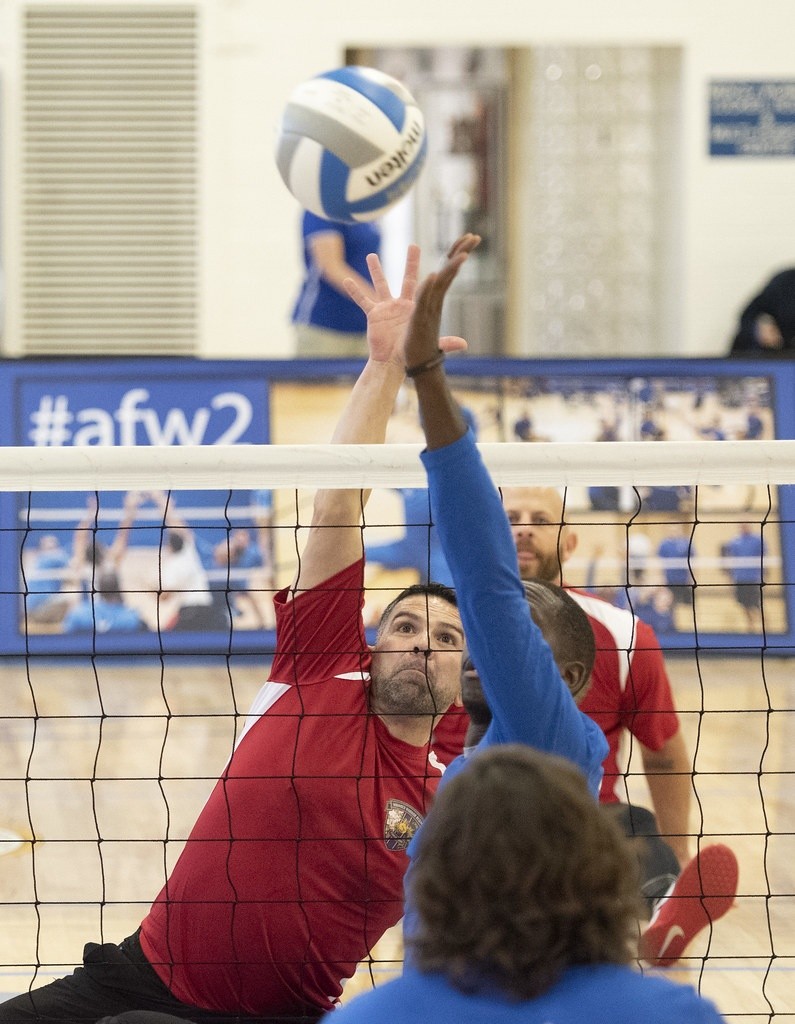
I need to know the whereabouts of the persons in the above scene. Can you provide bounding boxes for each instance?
[516,372,785,635]
[292,207,383,358]
[0,242,470,1024]
[321,745,726,1024]
[404,232,739,968]
[21,490,276,632]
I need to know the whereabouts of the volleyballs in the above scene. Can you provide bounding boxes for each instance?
[269,61,429,225]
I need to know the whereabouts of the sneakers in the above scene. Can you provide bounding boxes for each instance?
[637,843,738,970]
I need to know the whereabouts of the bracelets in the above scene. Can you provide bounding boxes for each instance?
[406,348,445,377]
